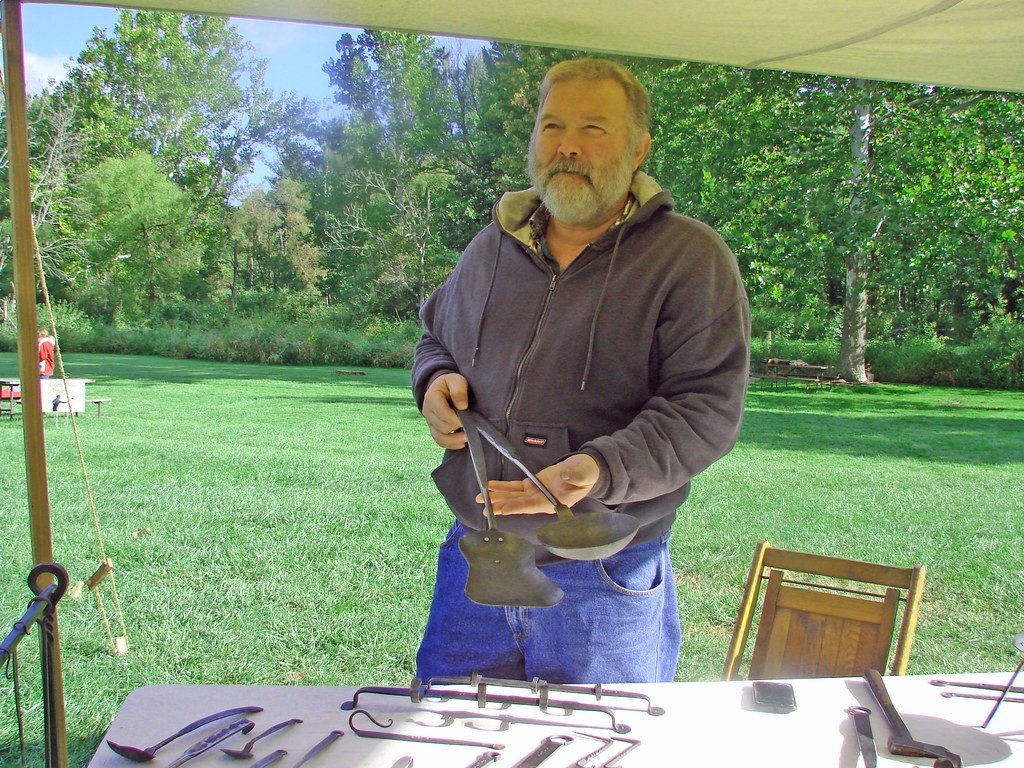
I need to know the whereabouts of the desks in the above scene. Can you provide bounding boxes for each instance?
[0,379,96,421]
[761,364,835,391]
[88,672,1024,768]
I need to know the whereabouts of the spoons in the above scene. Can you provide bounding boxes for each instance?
[220,719,303,759]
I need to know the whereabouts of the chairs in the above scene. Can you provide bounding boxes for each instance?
[724,537,927,680]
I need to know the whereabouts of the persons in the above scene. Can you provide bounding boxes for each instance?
[415,58,753,685]
[36,325,55,380]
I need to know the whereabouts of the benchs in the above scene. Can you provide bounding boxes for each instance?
[84,396,111,416]
[0,397,21,415]
[749,374,839,392]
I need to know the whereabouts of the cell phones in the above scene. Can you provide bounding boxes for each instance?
[753,681,797,712]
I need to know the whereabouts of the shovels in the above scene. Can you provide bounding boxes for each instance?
[456,407,566,609]
